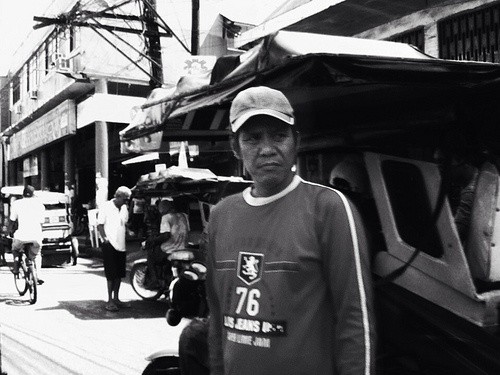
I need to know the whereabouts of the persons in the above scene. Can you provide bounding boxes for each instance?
[7,185,44,286]
[99,186,132,309]
[204,87,370,373]
[144,198,188,291]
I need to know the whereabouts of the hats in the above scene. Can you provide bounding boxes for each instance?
[229,86,295,133]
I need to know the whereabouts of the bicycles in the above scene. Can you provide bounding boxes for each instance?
[7,233,42,305]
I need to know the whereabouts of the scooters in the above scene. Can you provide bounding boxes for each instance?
[130,239,209,322]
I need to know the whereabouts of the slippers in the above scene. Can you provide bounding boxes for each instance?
[105,302,132,312]
